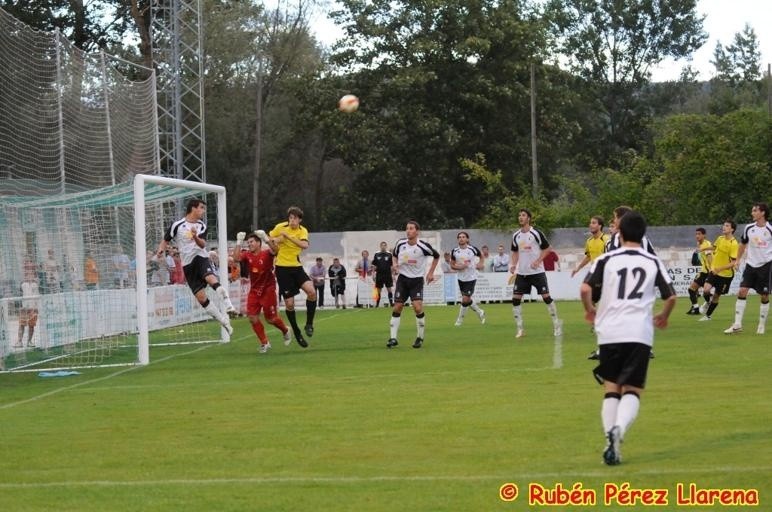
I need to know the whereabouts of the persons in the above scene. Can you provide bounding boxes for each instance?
[441,252,455,305]
[580,211,677,466]
[723,205,772,335]
[268,206,317,347]
[479,246,494,304]
[571,216,611,278]
[588,207,660,360]
[146,247,185,285]
[208,247,251,284]
[371,241,395,308]
[386,222,440,349]
[157,199,240,335]
[450,232,485,326]
[494,246,509,303]
[695,222,738,321]
[310,257,324,308]
[684,227,713,316]
[16,246,136,348]
[328,258,347,309]
[234,230,291,353]
[543,250,560,271]
[605,217,617,252]
[509,209,562,338]
[353,250,374,309]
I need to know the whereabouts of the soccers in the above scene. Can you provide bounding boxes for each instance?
[339,95,360,114]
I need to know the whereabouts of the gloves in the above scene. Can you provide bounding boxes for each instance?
[254,229,270,243]
[236,231,247,246]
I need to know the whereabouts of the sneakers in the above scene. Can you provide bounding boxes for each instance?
[294,330,308,347]
[724,324,744,334]
[386,338,399,348]
[12,341,24,347]
[699,301,711,314]
[304,324,314,337]
[222,319,234,336]
[757,324,765,335]
[699,315,712,321]
[515,329,526,338]
[226,305,236,314]
[553,318,564,336]
[259,340,272,354]
[26,341,35,347]
[479,310,486,324]
[412,337,424,348]
[283,326,292,346]
[602,424,622,466]
[686,306,700,315]
